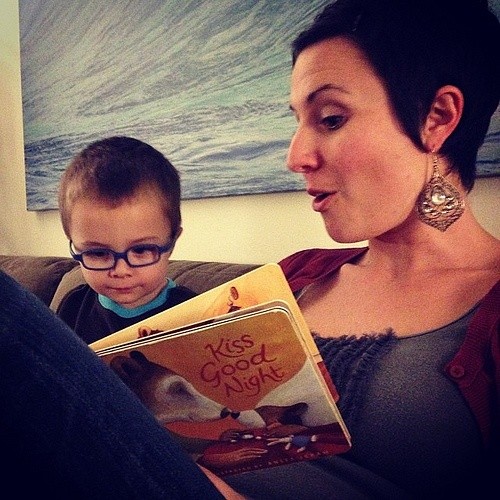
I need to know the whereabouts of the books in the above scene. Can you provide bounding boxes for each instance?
[86,262,352,478]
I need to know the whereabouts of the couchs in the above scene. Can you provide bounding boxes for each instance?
[0,252,267,351]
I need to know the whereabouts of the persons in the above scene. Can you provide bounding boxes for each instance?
[1,1,500,499]
[54,135,202,346]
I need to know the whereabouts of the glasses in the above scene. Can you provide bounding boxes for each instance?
[70,229,178,272]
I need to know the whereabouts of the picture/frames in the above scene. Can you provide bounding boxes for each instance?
[17,1,499,212]
